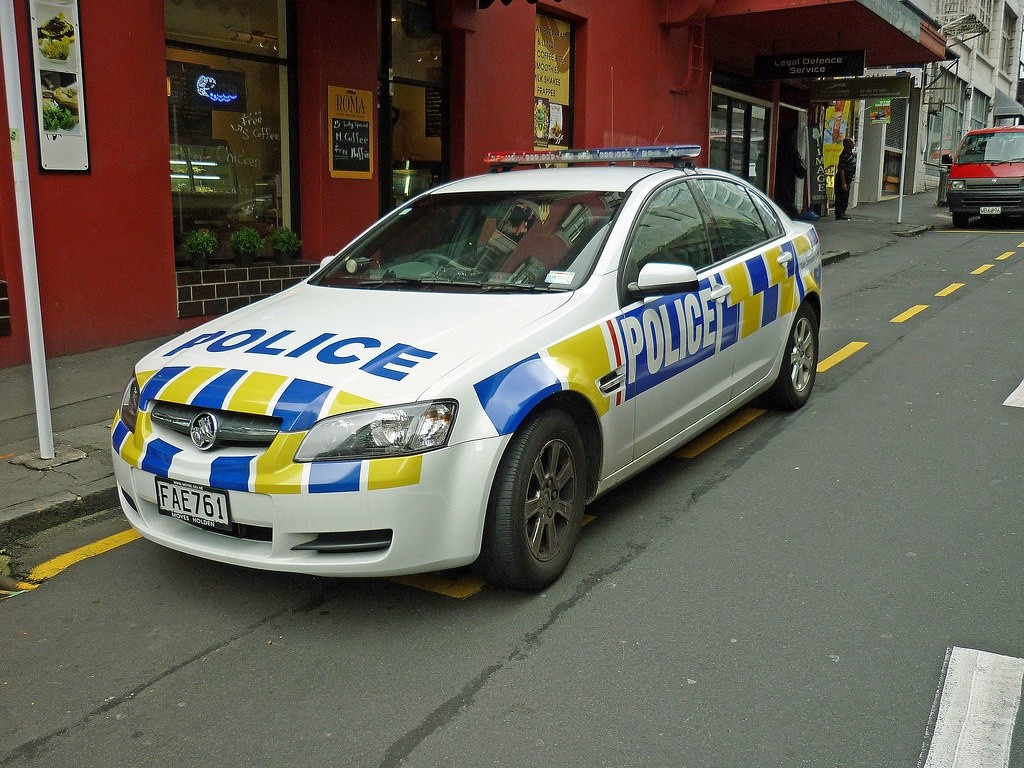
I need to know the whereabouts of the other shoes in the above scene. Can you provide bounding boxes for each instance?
[835,213,851,220]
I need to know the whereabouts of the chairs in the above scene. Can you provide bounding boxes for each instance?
[975,140,990,155]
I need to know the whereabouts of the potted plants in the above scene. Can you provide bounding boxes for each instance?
[266,225,306,265]
[181,228,219,269]
[227,226,265,269]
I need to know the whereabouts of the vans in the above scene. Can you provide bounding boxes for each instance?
[942,125,1024,228]
[932,137,951,159]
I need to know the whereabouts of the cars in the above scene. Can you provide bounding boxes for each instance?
[108,142,823,594]
[731,144,761,181]
[226,194,282,226]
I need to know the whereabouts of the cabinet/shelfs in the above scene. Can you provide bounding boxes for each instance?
[169,135,243,213]
[253,178,277,222]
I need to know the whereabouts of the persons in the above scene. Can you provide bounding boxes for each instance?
[775,130,807,219]
[835,139,856,219]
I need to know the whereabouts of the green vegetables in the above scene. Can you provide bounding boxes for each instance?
[42,104,76,130]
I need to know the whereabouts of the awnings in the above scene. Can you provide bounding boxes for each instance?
[994,89,1024,119]
